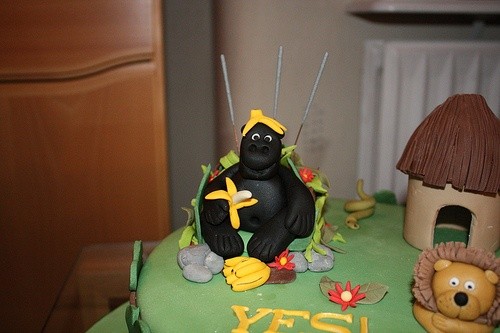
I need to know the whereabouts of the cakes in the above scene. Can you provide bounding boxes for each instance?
[84,93,500,333]
